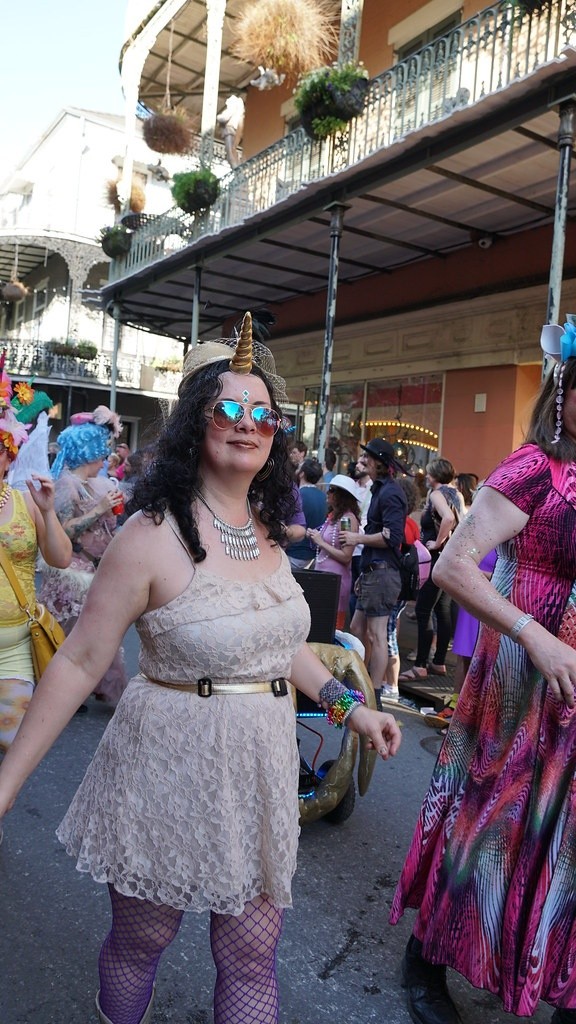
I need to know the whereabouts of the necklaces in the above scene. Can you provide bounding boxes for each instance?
[0,483,11,508]
[194,489,260,560]
[317,520,337,563]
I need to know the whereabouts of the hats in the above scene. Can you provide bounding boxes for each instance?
[315,474,363,503]
[358,439,395,469]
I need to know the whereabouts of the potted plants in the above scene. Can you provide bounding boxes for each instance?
[142,359,183,395]
[169,168,221,214]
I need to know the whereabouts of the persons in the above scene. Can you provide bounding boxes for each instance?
[0,336,400,1024]
[391,313,576,1024]
[217,95,244,168]
[0,397,73,755]
[278,437,498,711]
[6,376,146,712]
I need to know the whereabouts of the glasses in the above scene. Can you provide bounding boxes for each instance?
[202,401,281,437]
[329,486,336,493]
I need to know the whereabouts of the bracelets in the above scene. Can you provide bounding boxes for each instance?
[510,614,534,643]
[317,678,366,729]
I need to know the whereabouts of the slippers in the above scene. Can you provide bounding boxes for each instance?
[426,664,447,676]
[398,668,428,682]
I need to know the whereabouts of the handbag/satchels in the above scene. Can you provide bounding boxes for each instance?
[29,602,66,684]
[399,542,420,601]
[428,490,462,556]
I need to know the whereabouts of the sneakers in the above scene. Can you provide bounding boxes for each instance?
[424,707,454,729]
[380,688,399,704]
[407,647,436,660]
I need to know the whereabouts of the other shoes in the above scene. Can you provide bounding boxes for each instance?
[76,704,88,717]
[406,611,417,623]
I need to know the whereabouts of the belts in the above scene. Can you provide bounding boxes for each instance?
[140,671,288,698]
[362,562,392,575]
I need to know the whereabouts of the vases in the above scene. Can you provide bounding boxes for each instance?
[101,233,131,258]
[299,79,367,138]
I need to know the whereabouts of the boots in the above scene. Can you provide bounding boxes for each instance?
[551,1007,576,1024]
[96,982,156,1024]
[401,933,462,1024]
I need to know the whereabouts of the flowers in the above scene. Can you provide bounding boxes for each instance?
[0,428,18,458]
[13,382,36,405]
[96,224,129,243]
[293,55,369,112]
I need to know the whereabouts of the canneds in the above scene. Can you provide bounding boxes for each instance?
[340,517,351,532]
[106,490,124,516]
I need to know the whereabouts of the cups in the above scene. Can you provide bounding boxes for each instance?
[108,490,125,515]
